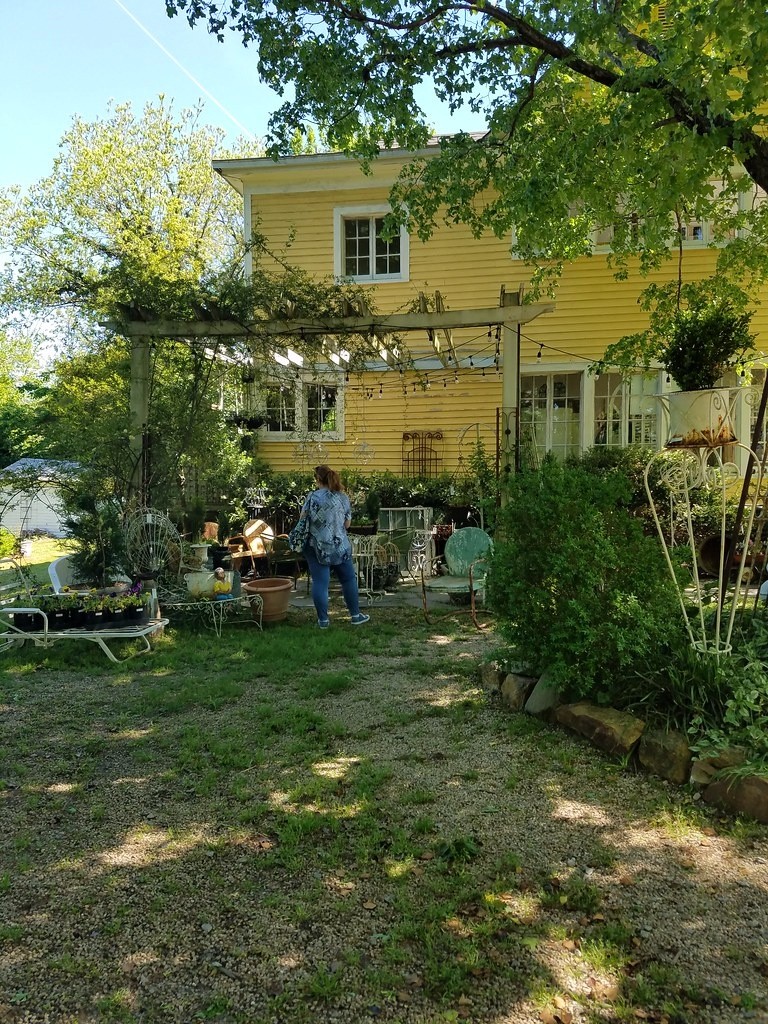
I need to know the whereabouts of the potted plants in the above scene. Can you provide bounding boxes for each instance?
[586,274,763,444]
[213,510,235,570]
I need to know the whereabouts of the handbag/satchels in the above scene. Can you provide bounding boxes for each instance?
[287,490,314,553]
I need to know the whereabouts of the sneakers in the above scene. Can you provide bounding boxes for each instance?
[318,618,329,629]
[351,613,370,625]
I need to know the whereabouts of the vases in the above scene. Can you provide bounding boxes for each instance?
[244,578,295,621]
[15,605,149,632]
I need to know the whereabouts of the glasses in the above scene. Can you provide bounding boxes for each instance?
[312,466,323,480]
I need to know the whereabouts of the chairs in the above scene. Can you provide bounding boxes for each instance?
[122,505,264,641]
[422,526,500,630]
[0,558,170,663]
[377,526,415,583]
[259,531,311,594]
[242,519,302,577]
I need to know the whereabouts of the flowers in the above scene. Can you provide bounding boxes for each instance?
[13,582,152,614]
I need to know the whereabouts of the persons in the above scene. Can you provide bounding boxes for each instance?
[286,464,372,628]
[214,567,235,613]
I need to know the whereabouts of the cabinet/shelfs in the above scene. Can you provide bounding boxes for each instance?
[377,507,435,578]
[346,533,384,605]
[643,387,762,670]
[404,433,444,478]
